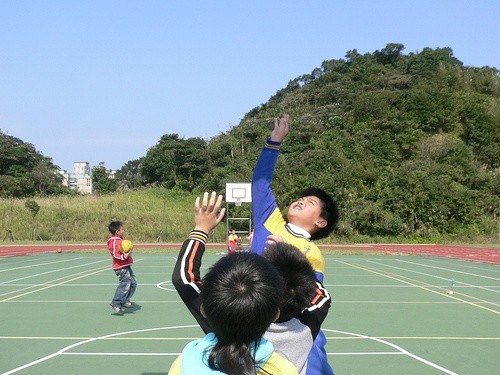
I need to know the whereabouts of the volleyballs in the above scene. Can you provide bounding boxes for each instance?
[119,239,134,254]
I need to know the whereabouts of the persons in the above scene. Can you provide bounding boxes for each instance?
[107,221,137,315]
[166,110,340,375]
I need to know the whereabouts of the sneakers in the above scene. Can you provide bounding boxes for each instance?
[122,302,134,308]
[110,303,124,315]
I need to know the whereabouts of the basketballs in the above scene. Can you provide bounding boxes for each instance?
[234,238,240,243]
[57,248,62,253]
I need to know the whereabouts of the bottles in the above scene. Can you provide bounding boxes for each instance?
[449,278,455,295]
